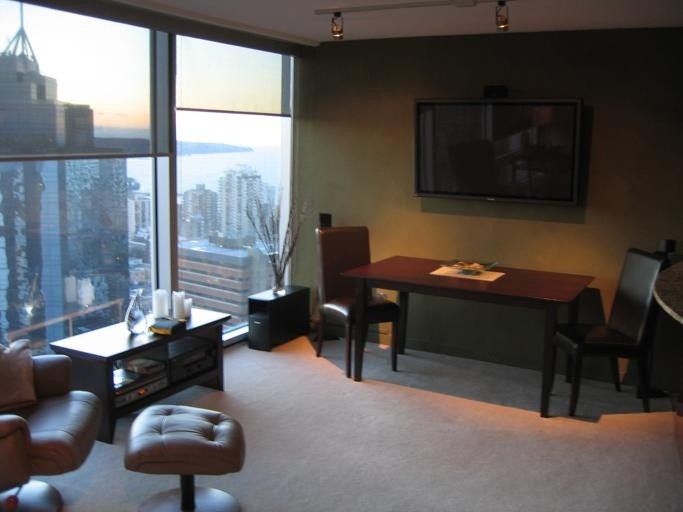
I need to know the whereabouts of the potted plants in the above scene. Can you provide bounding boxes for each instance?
[245,194,307,296]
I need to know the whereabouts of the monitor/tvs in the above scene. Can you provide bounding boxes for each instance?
[413,97,591,208]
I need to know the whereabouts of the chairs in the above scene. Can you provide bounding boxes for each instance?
[314,226,401,378]
[0,353,104,511]
[549,246,669,417]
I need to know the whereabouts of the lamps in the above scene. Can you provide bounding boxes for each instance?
[495,1,509,32]
[330,11,343,39]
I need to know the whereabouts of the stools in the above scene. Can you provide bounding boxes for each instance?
[123,403,246,512]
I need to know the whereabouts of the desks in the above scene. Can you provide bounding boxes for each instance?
[338,255,595,418]
[48,308,232,444]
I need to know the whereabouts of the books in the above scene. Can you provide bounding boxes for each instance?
[148,318,182,335]
[126,357,165,376]
